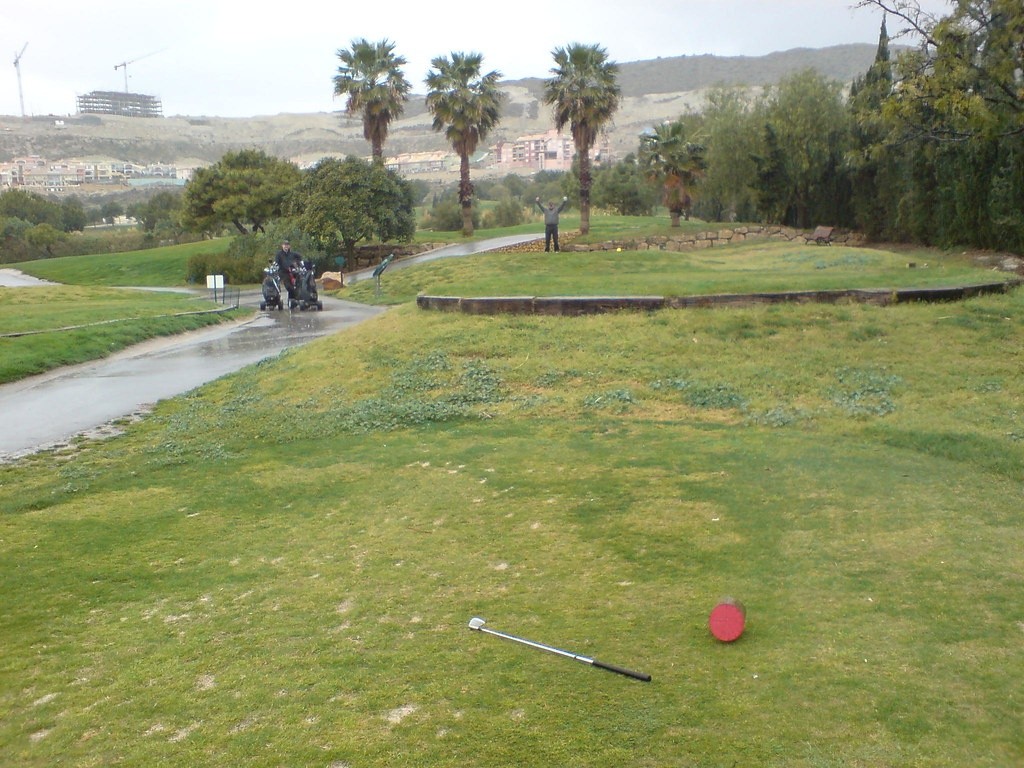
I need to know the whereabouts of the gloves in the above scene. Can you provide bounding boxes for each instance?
[536,197,539,202]
[563,197,568,201]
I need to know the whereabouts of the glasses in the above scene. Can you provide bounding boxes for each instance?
[283,242,289,245]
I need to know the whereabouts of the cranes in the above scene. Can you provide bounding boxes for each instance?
[13,40,29,116]
[116,57,135,90]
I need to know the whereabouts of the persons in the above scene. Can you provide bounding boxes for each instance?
[274,240,303,309]
[535,196,568,254]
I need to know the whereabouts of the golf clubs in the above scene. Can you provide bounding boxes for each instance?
[468,617,651,682]
